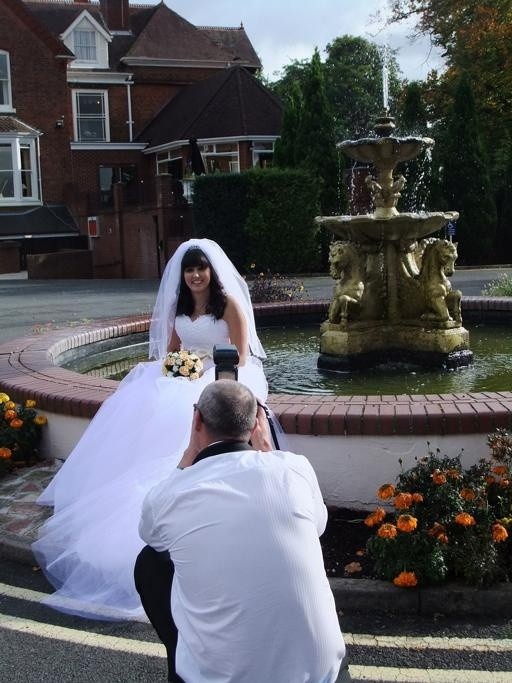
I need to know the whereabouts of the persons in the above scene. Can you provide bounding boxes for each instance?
[31,236,270,619]
[134,378,354,683]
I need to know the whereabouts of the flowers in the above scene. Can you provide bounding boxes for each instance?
[0,391,49,478]
[364,423,512,589]
[162,348,206,381]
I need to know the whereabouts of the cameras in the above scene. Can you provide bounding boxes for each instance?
[212,345,239,381]
